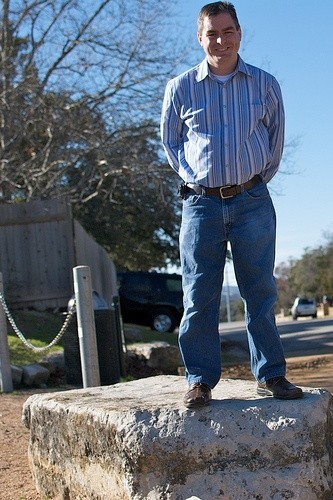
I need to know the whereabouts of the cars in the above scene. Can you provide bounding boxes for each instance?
[291,297,317,320]
[116,270,185,334]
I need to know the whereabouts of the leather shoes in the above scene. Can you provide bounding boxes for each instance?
[183,383,212,409]
[257,375,303,400]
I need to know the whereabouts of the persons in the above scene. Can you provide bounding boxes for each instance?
[160,1,304,409]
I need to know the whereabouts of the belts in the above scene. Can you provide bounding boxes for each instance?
[186,174,263,199]
